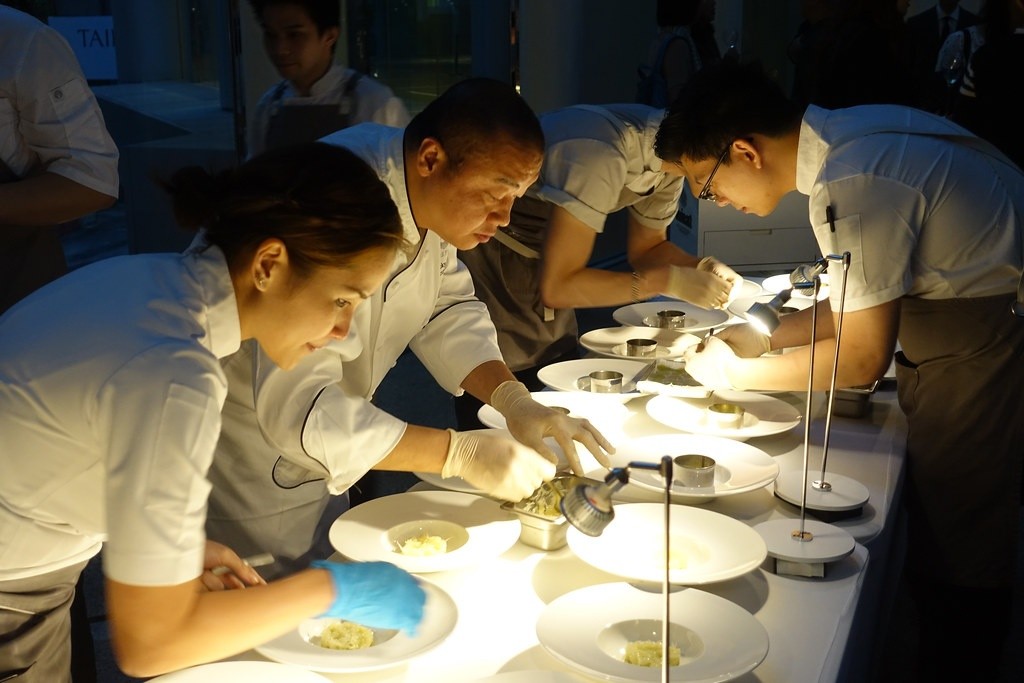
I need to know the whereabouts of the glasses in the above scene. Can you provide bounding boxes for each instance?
[698,137,754,202]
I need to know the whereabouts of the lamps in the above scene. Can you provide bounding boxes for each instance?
[790,250,851,491]
[745,276,821,543]
[561,456,673,683]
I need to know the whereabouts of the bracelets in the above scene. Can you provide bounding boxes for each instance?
[631,271,640,303]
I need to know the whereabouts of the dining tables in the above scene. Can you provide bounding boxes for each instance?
[224,538,867,683]
[589,384,910,538]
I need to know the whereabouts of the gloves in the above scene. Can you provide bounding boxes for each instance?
[312,559,425,636]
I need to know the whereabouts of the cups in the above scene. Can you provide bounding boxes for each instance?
[706,403,745,430]
[626,339,658,357]
[548,406,570,416]
[588,370,623,394]
[672,454,716,487]
[779,307,799,318]
[656,310,686,328]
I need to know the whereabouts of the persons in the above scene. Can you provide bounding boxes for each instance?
[786,0,1024,171]
[0,140,429,683]
[243,0,412,159]
[187,81,618,585]
[0,3,120,321]
[650,0,749,112]
[455,104,744,435]
[653,73,1024,683]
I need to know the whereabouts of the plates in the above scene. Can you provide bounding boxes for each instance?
[536,582,770,683]
[730,279,762,315]
[254,573,459,674]
[762,273,830,301]
[537,358,672,404]
[143,660,331,683]
[328,489,522,572]
[646,389,802,442]
[413,438,602,494]
[565,500,768,587]
[579,326,702,363]
[612,301,730,334]
[604,433,781,503]
[478,390,630,436]
